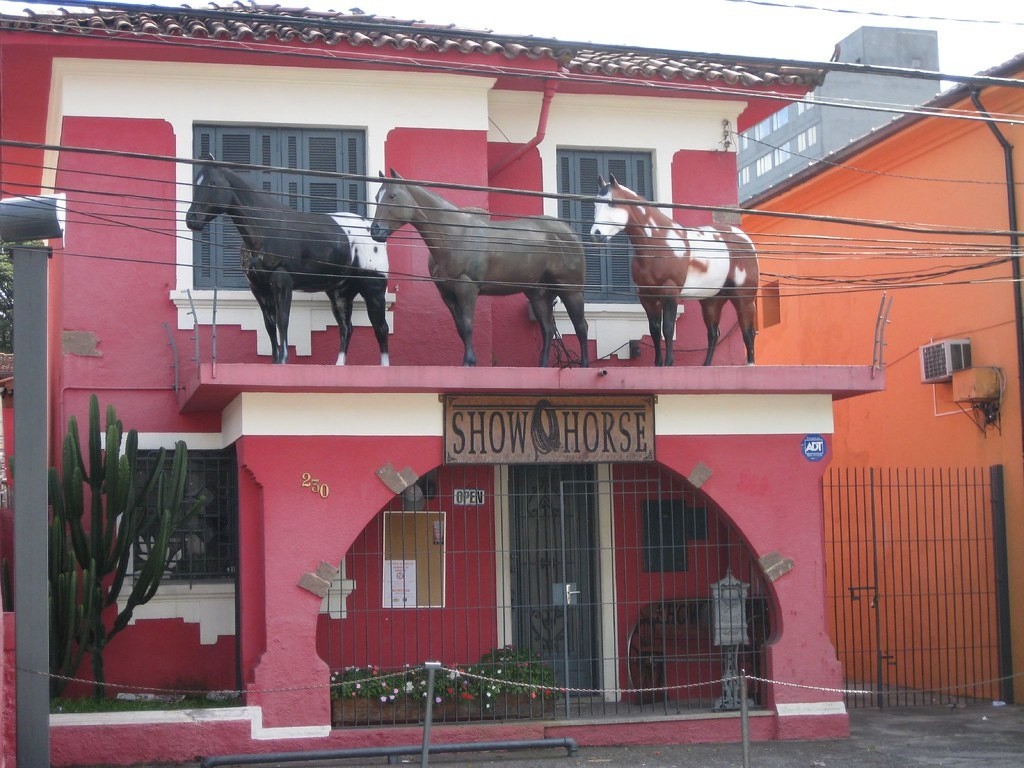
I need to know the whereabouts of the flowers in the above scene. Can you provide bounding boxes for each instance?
[331,645,567,713]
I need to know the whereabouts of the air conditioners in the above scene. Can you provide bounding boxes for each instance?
[919,339,971,384]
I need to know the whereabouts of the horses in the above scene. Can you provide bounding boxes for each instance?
[371,168,589,366]
[185,152,391,366]
[591,173,760,366]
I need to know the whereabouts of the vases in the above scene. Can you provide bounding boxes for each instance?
[333,692,557,725]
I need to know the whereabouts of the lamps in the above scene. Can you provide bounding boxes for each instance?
[710,567,755,711]
